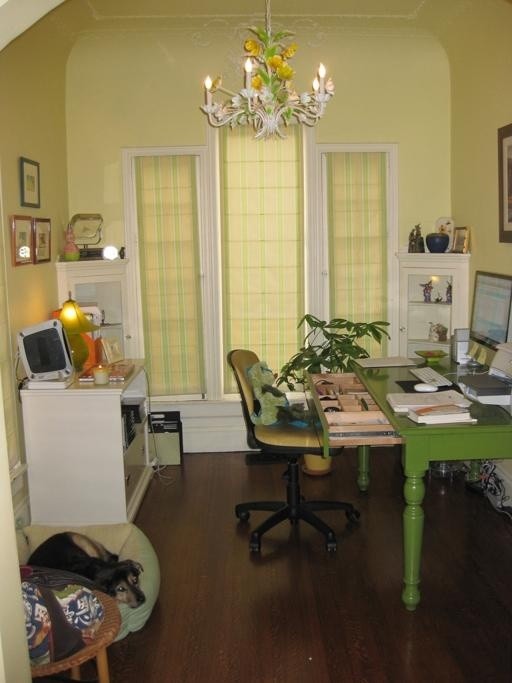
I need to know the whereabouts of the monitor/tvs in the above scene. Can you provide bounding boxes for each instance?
[470,270,512,374]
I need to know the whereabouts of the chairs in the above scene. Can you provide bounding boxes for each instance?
[222,344,363,563]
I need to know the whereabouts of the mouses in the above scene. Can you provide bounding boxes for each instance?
[414,383,438,392]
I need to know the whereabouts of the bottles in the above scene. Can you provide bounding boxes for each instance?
[62,219,82,264]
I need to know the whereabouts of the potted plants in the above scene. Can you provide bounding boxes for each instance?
[273,310,392,476]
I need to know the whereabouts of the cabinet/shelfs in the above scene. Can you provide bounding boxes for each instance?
[52,257,134,361]
[16,359,156,527]
[391,250,471,363]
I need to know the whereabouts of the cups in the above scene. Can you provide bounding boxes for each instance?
[425,231,449,253]
[91,365,114,386]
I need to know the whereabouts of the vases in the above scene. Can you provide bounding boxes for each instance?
[424,232,449,252]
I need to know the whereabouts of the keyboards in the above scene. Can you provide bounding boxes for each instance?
[409,367,453,387]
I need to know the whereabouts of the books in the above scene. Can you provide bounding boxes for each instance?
[407,404,470,423]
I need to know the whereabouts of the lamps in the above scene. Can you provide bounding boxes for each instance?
[196,0,340,141]
[54,288,102,371]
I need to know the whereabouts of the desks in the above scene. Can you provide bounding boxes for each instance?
[30,590,122,682]
[300,349,512,612]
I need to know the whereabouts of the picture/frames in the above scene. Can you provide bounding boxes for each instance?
[34,216,52,263]
[17,153,44,208]
[8,211,36,268]
[450,224,472,254]
[493,120,512,244]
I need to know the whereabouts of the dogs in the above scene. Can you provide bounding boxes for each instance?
[26,531,146,610]
